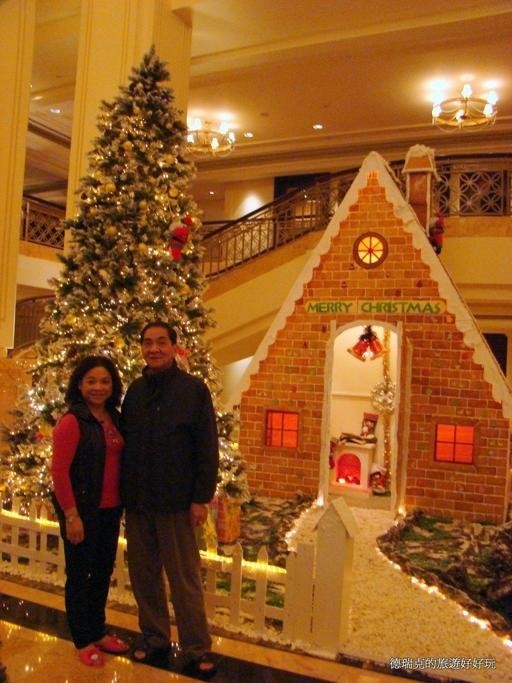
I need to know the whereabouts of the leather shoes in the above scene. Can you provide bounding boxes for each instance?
[72,633,131,667]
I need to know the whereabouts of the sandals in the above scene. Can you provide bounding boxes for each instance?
[131,638,172,662]
[188,651,216,679]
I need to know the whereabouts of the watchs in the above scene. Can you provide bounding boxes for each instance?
[64,510,79,524]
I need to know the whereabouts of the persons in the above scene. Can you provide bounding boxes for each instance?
[48,354,131,671]
[116,320,221,677]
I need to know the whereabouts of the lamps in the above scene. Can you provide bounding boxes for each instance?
[423,78,507,133]
[170,110,255,169]
